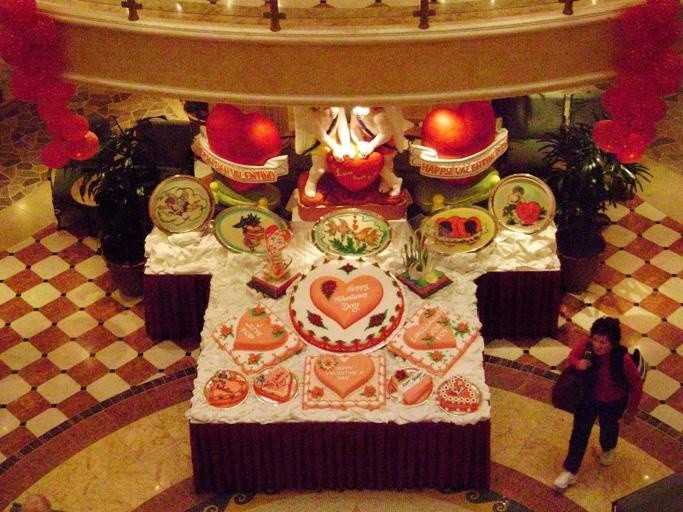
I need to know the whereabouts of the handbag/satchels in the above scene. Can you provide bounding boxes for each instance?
[631,349,648,382]
[552,364,590,413]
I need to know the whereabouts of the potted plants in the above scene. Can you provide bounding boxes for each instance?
[540,117,654,294]
[64,114,167,296]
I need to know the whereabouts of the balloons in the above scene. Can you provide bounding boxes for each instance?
[591,0,683,165]
[0,0,101,170]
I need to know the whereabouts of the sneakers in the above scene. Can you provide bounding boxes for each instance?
[554,471,578,488]
[600,449,614,466]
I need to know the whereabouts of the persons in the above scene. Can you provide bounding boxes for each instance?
[293,105,356,198]
[159,191,203,214]
[553,317,644,489]
[349,105,413,197]
[506,186,525,226]
